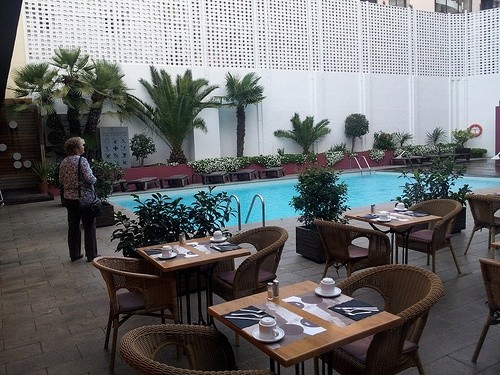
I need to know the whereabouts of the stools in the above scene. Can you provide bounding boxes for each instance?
[158,174,191,189]
[230,168,256,183]
[259,166,286,179]
[127,175,159,190]
[202,171,228,184]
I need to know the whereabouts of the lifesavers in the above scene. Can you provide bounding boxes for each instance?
[467,124,482,137]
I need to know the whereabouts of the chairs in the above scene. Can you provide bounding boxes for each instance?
[214,224,290,346]
[472,257,500,364]
[89,253,192,375]
[117,323,274,375]
[393,197,464,278]
[464,191,500,258]
[318,262,445,375]
[312,219,391,285]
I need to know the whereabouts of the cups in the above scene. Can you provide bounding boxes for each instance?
[259,320,279,339]
[321,278,335,294]
[380,212,387,220]
[397,203,405,209]
[162,246,172,257]
[213,231,222,240]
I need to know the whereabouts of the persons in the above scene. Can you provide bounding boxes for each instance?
[59,137,101,262]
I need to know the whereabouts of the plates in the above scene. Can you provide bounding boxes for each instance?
[377,218,392,221]
[210,237,227,242]
[158,252,177,258]
[314,286,342,297]
[394,208,408,212]
[252,326,285,342]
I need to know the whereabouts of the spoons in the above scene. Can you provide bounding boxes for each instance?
[239,308,265,313]
[345,311,371,315]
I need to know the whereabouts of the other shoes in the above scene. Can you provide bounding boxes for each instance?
[71,254,83,261]
[88,255,101,262]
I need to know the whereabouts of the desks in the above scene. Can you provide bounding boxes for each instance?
[344,210,445,266]
[207,279,402,375]
[133,235,254,344]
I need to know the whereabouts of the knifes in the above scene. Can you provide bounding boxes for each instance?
[334,307,377,309]
[225,316,261,320]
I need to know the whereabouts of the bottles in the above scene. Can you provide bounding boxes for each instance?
[267,283,274,300]
[179,234,183,247]
[273,280,280,298]
[182,234,185,244]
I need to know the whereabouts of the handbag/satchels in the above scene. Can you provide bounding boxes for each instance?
[81,194,102,217]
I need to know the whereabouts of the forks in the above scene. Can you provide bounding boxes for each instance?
[342,308,380,312]
[231,313,267,317]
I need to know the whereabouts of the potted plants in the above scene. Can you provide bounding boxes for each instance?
[88,158,123,228]
[30,158,58,192]
[397,149,471,234]
[290,167,354,263]
[112,186,235,303]
